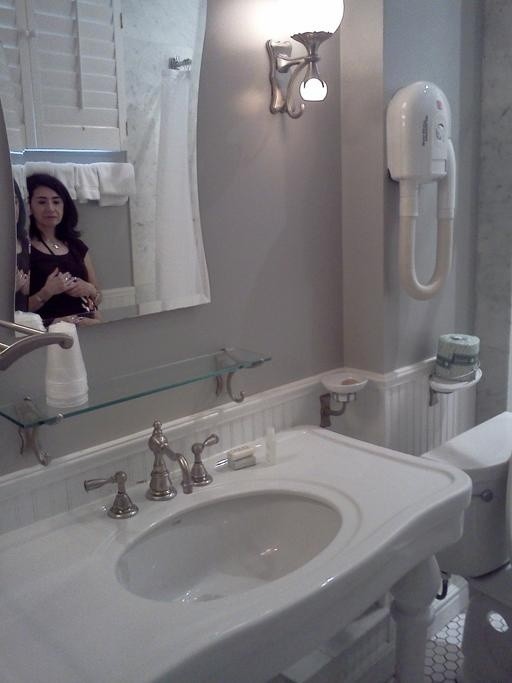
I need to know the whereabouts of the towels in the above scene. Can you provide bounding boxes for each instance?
[12,160,136,208]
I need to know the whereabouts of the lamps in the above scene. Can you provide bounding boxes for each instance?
[265,0,345,118]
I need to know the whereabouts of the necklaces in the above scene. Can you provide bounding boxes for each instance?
[47,237,60,249]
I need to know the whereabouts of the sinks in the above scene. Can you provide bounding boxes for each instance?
[94,477,358,622]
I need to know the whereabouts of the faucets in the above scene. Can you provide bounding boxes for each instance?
[143,418,193,499]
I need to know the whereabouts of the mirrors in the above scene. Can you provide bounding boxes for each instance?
[9,148,141,340]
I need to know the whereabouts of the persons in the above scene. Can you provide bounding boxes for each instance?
[12,180,28,308]
[28,171,101,313]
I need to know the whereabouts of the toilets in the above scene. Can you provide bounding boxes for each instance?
[420,411,512,683]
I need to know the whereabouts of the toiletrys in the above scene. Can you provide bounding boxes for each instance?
[264,425,278,465]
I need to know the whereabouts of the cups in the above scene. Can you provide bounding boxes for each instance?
[44,322,89,408]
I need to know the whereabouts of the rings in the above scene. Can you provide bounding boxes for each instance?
[24,273,27,276]
[65,276,68,280]
[21,274,23,277]
[75,276,77,280]
